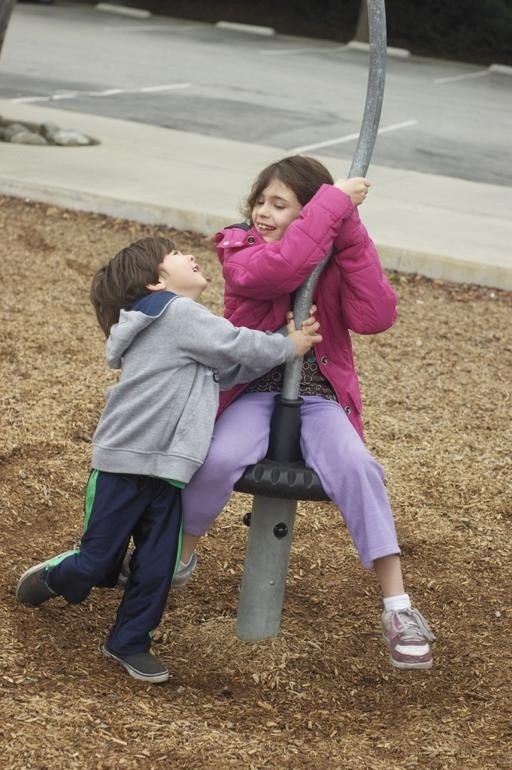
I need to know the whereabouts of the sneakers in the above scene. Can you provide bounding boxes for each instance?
[381,607,435,670]
[114,554,197,587]
[102,645,168,683]
[16,550,79,605]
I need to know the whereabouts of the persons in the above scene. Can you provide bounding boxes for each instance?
[111,153,437,672]
[14,236,322,684]
